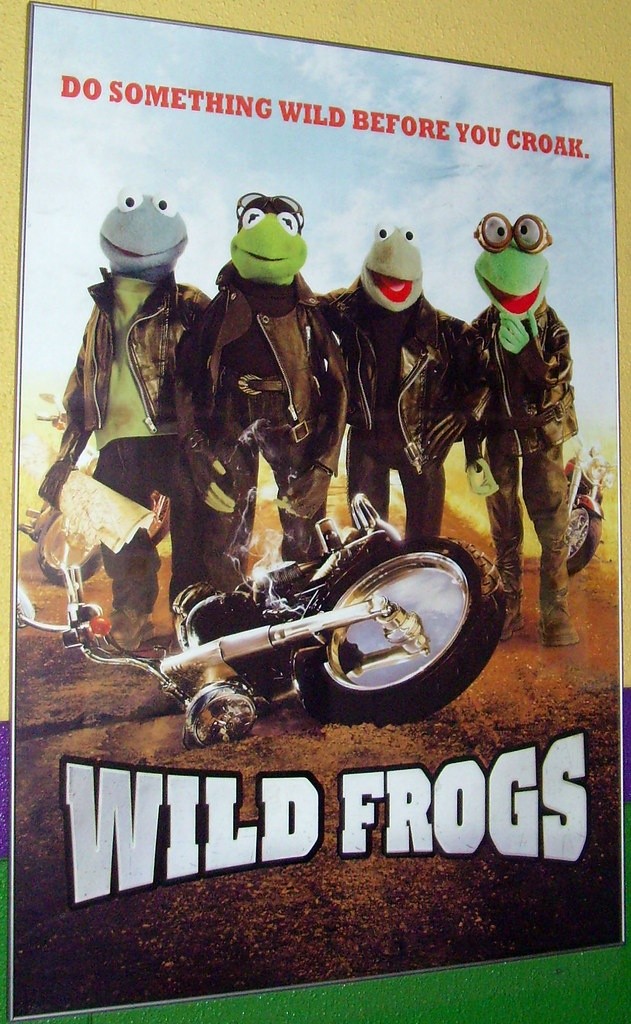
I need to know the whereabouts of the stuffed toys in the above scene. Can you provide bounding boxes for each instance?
[32,182,600,654]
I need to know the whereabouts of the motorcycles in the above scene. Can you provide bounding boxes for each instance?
[17,391,173,586]
[563,429,615,580]
[16,492,510,750]
